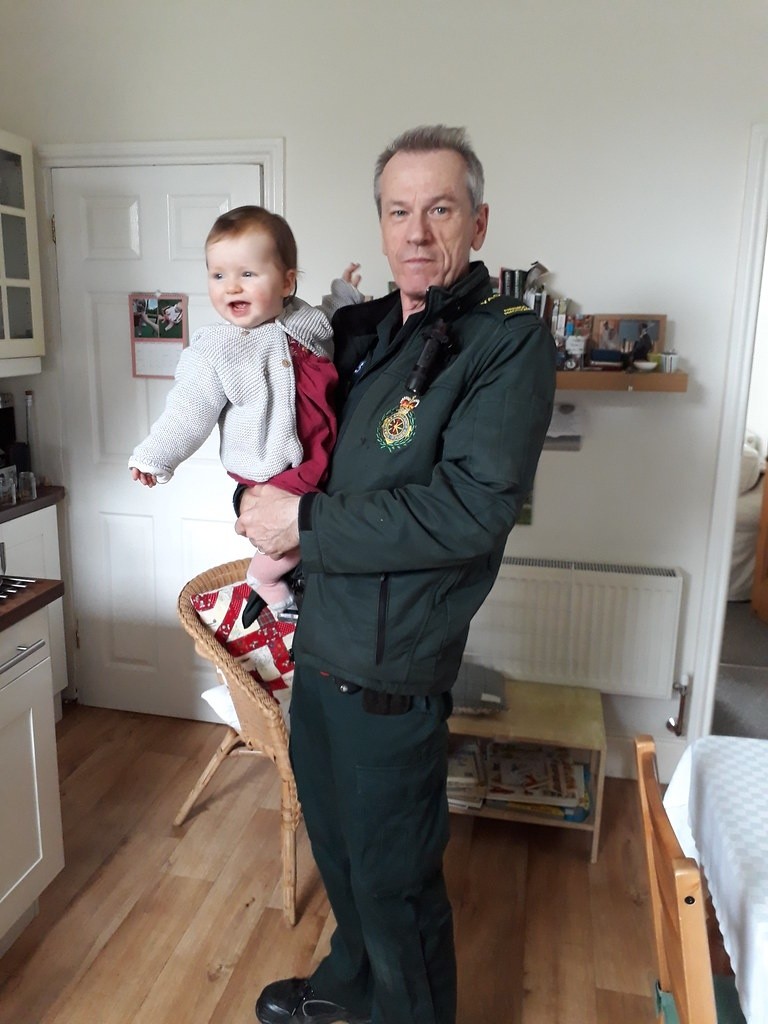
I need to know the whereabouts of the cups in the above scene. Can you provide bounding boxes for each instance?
[0,478,16,507]
[19,471,37,500]
[659,352,678,374]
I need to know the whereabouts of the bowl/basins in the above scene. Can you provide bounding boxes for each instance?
[633,360,658,371]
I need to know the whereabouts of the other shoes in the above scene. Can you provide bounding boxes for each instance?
[255,977,371,1024]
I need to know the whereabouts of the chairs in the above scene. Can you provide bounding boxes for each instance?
[633,734,747,1024]
[173,558,304,927]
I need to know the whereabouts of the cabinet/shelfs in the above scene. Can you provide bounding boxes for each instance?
[446,681,606,863]
[0,129,45,378]
[0,580,65,941]
[0,487,68,723]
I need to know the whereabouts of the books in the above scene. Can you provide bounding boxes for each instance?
[448,741,590,822]
[498,264,580,369]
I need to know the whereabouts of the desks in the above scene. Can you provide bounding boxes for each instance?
[662,735,768,1024]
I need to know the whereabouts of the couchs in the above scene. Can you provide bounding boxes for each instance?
[724,429,768,601]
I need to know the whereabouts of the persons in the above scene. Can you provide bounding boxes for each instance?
[127,206,362,624]
[234,127,558,1024]
[602,320,652,356]
[133,299,182,337]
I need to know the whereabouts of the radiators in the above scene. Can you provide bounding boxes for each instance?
[463,557,683,698]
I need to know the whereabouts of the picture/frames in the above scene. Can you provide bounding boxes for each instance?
[593,313,666,363]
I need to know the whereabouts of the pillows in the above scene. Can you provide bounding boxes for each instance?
[448,664,509,714]
[190,581,299,740]
